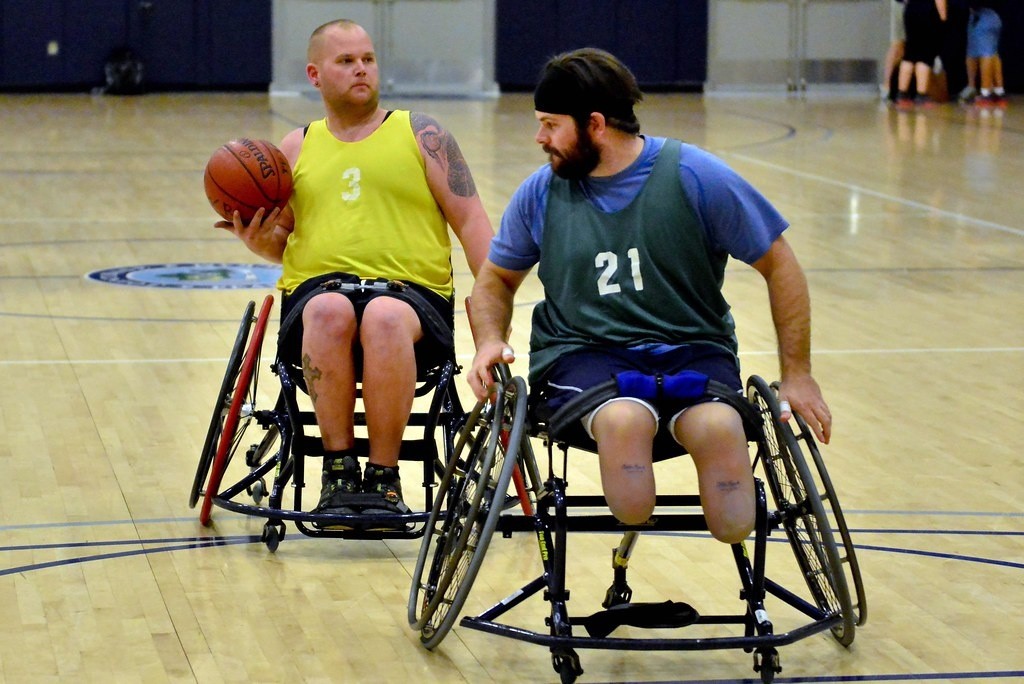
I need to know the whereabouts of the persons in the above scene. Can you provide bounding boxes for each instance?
[470,48,833,543]
[879,0,1007,108]
[213,20,512,532]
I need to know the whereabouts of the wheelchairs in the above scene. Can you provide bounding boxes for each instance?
[188,295,536,553]
[407,361,868,684]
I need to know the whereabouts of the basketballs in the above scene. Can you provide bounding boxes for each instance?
[204,138,292,221]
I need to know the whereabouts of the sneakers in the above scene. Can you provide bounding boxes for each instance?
[887,92,1007,105]
[316,453,410,532]
